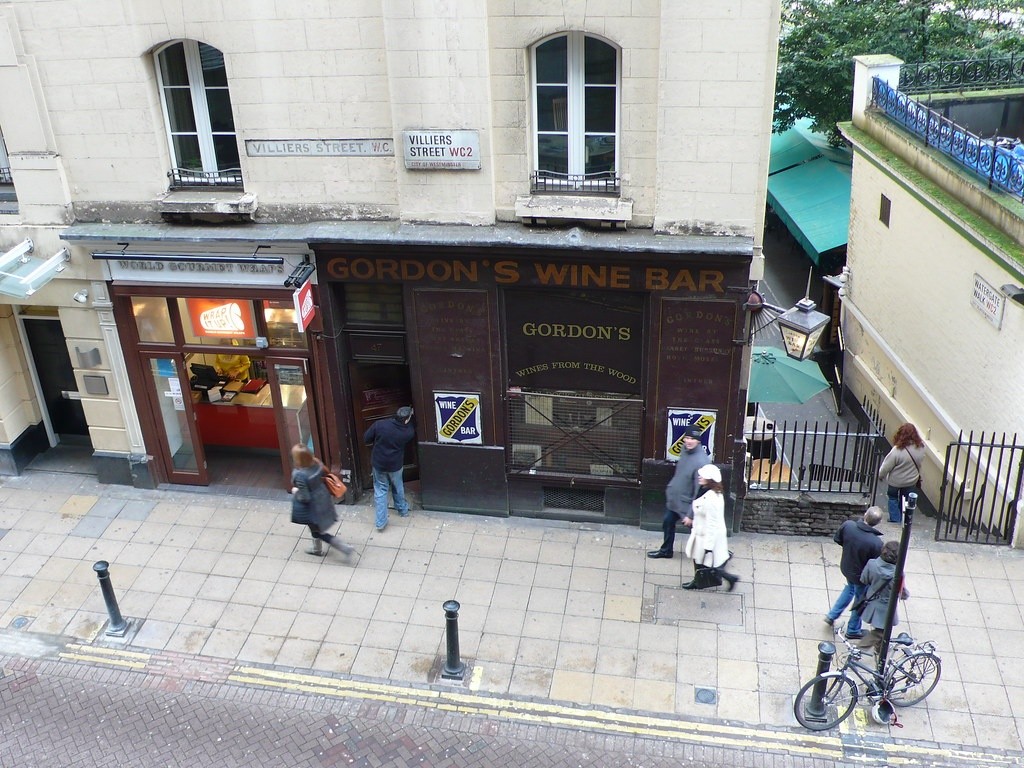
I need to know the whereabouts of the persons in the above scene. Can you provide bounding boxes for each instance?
[879,423,926,522]
[823,505,885,638]
[860,542,910,655]
[647,424,711,558]
[683,464,739,592]
[291,443,361,565]
[214,337,251,385]
[363,406,416,528]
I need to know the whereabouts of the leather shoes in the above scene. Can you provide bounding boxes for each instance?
[726,575,739,592]
[647,551,672,558]
[682,580,701,590]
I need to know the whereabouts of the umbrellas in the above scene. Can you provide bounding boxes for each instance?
[748,345,831,430]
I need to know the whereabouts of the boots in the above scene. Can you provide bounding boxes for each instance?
[328,538,355,562]
[305,536,323,556]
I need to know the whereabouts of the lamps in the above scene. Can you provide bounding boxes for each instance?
[732,267,831,362]
[73,289,88,303]
[1000,283,1024,307]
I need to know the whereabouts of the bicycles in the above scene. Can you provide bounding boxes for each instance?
[794,622,946,732]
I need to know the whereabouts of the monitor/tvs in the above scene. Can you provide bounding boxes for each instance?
[190,363,218,381]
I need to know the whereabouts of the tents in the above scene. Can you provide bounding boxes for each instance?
[766,91,853,267]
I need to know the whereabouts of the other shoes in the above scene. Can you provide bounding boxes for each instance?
[824,618,834,626]
[875,644,893,655]
[843,629,869,639]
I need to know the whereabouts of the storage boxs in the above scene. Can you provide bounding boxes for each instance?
[207,388,222,402]
[191,390,202,403]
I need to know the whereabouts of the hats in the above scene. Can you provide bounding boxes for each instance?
[683,424,704,442]
[698,463,723,483]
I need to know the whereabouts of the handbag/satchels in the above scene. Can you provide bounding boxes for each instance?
[313,458,346,500]
[850,595,870,616]
[695,551,721,590]
[917,475,922,489]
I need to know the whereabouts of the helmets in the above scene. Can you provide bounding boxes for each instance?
[872,700,895,724]
